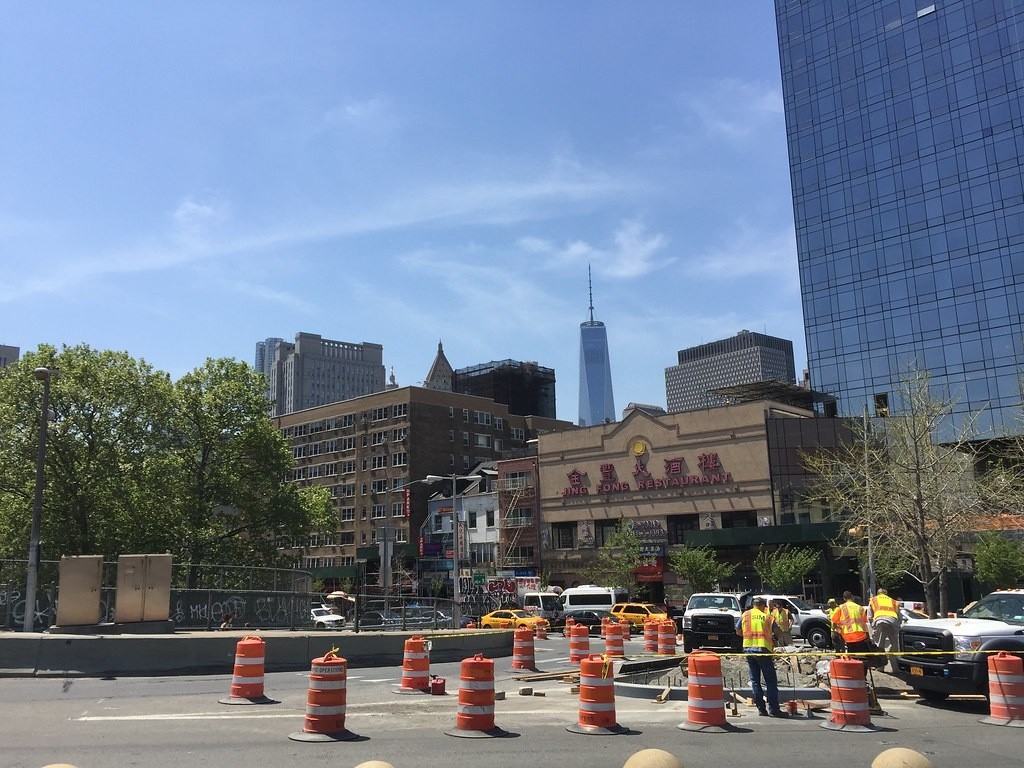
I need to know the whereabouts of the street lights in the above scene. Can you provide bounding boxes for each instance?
[22,367,55,633]
[381,479,434,619]
[801,575,812,604]
[427,473,483,629]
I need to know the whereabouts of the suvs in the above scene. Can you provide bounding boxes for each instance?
[671,591,753,654]
[893,587,1024,703]
[610,602,669,635]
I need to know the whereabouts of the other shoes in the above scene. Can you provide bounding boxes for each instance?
[893,669,902,673]
[875,668,884,673]
[759,711,769,716]
[769,711,789,717]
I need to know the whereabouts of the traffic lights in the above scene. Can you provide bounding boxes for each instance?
[848,526,863,537]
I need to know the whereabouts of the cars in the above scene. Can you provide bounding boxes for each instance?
[347,600,476,631]
[477,608,551,636]
[554,608,637,637]
[860,603,925,640]
[311,608,347,631]
[310,600,342,615]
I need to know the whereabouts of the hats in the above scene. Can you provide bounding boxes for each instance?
[753,598,767,607]
[828,599,836,603]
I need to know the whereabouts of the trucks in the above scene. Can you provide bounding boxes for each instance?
[459,573,566,633]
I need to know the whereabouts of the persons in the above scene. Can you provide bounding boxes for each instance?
[734,597,794,719]
[820,588,902,674]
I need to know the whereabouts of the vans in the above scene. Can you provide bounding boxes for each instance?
[555,583,626,616]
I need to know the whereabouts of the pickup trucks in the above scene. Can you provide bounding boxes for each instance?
[671,591,832,649]
